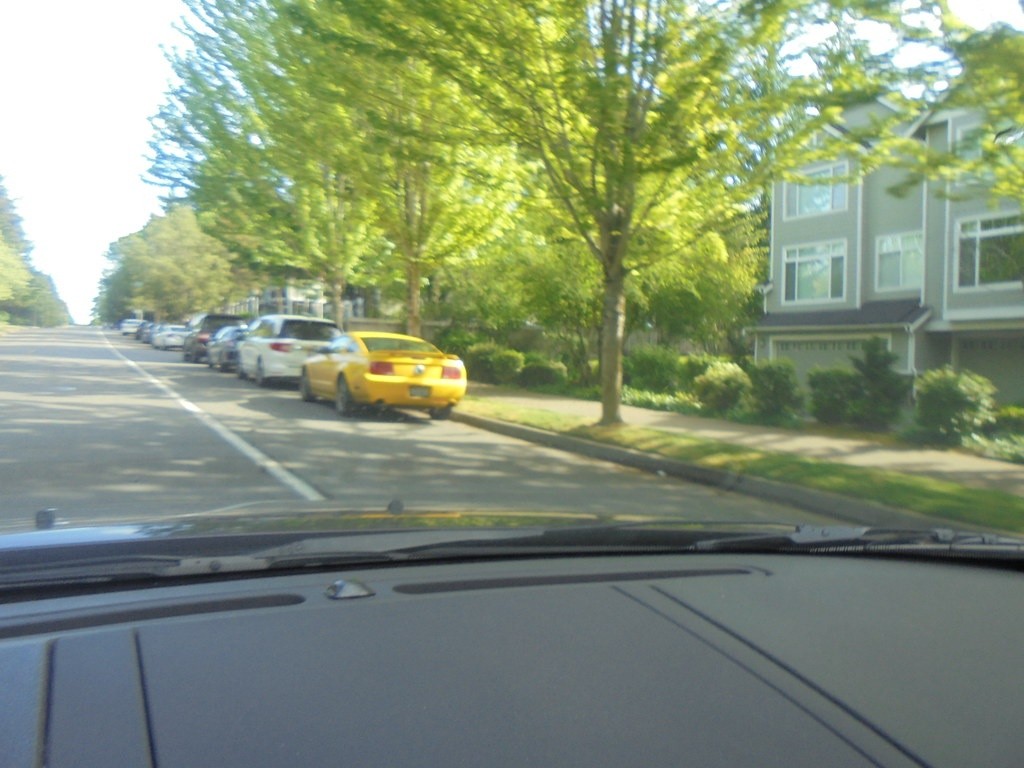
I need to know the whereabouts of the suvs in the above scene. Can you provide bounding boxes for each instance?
[182,312,244,364]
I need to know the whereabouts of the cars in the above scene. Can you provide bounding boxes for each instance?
[205,324,248,372]
[120,320,139,336]
[236,313,345,385]
[299,330,468,419]
[135,321,191,351]
